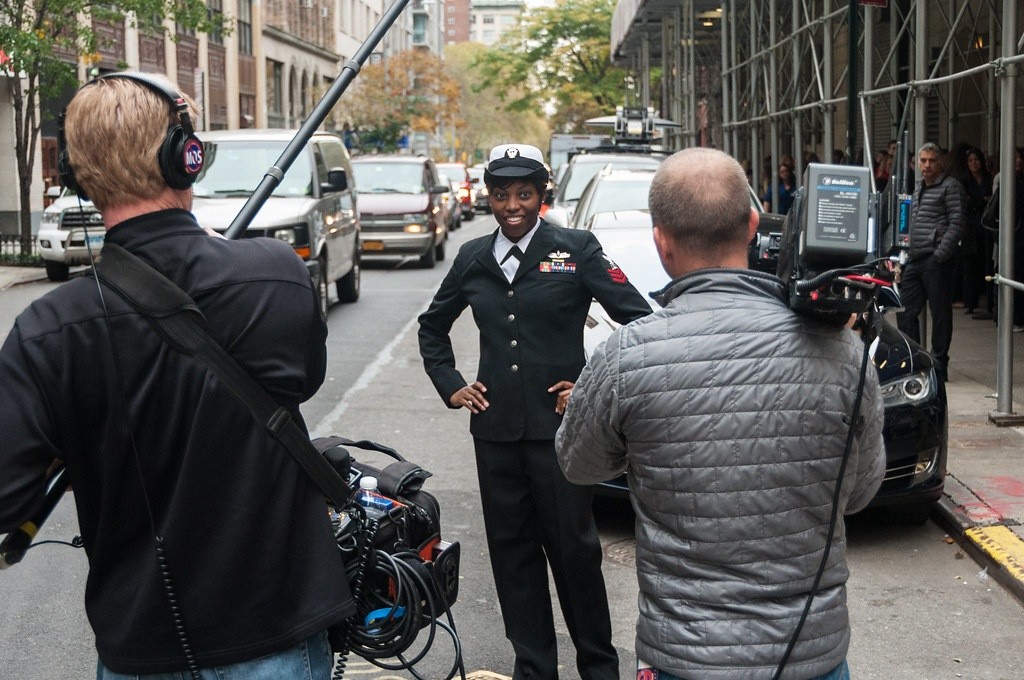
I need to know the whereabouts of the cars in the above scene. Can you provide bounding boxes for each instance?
[539,128,947,534]
[433,161,490,232]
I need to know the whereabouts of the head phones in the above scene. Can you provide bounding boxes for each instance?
[57,72,204,202]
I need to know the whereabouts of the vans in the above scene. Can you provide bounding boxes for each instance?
[187,126,363,323]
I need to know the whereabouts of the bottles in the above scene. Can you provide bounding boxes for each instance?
[355,476,387,519]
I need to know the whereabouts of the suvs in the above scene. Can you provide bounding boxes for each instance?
[348,154,447,268]
[35,176,109,282]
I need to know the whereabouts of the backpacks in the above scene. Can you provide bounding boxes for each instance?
[981,179,1024,233]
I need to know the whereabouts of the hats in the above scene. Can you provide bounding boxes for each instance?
[484,144,549,189]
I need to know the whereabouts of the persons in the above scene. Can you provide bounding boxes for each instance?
[0,69,356,680]
[896,144,969,381]
[417,144,657,680]
[555,147,885,680]
[736,146,1024,333]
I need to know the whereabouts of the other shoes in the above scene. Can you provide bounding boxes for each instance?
[953,302,1024,333]
